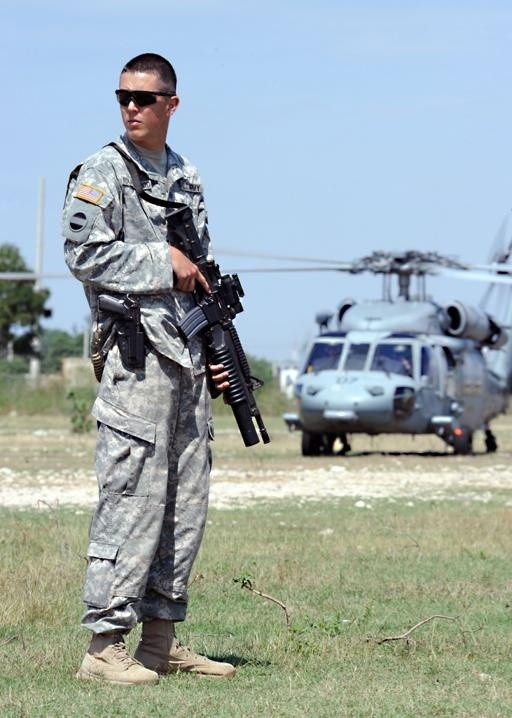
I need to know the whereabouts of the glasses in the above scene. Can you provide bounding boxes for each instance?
[115,89,175,107]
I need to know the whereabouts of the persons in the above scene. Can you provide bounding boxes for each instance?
[60,52,237,683]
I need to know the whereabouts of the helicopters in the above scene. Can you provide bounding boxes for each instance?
[0,247,512,455]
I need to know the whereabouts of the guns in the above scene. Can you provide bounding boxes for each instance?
[99,294,145,368]
[166,205,271,448]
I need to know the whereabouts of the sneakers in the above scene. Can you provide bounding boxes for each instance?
[131,622,238,681]
[75,632,160,686]
